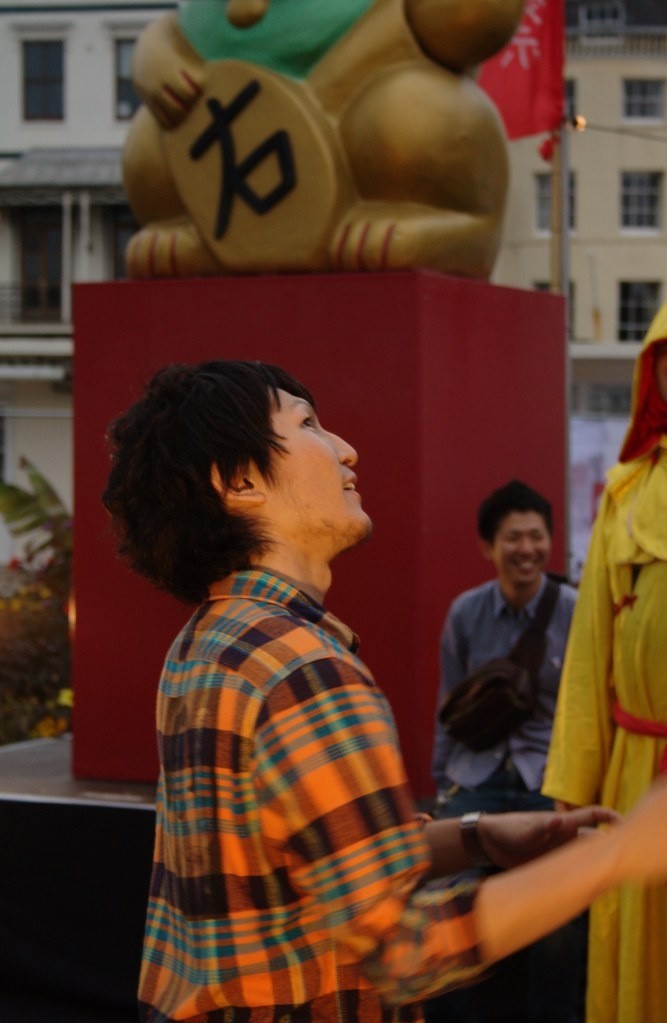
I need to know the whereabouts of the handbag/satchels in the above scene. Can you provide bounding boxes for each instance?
[437,658,538,753]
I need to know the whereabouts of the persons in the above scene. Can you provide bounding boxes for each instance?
[102,360,667,1023]
[543,299,667,1023]
[424,477,576,1023]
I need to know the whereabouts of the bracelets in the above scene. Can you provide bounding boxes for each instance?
[460,811,491,867]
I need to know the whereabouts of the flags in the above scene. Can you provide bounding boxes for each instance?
[476,0,566,143]
